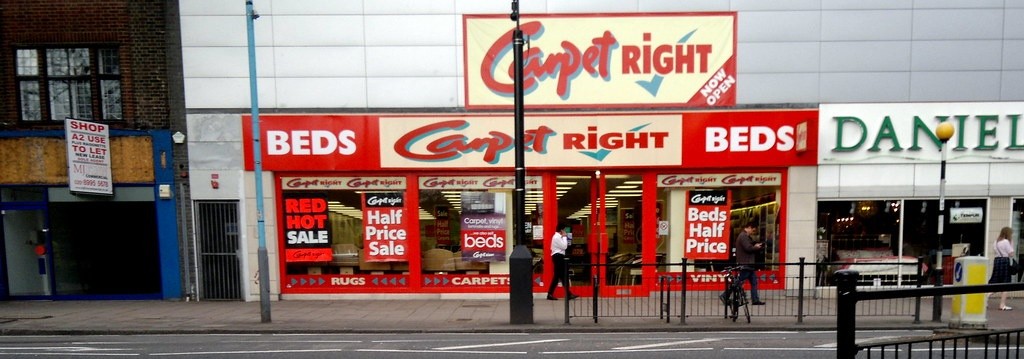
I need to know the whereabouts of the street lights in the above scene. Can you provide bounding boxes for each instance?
[931,121,955,321]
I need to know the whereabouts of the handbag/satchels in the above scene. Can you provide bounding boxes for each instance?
[1007,258,1019,275]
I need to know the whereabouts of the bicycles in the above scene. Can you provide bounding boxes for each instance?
[722,265,751,323]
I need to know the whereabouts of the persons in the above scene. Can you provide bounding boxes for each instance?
[988,227,1015,310]
[718,222,766,305]
[547,223,580,300]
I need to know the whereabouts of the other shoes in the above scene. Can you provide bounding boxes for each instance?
[752,298,765,304]
[719,293,730,306]
[547,292,558,300]
[568,292,579,300]
[998,305,1012,310]
